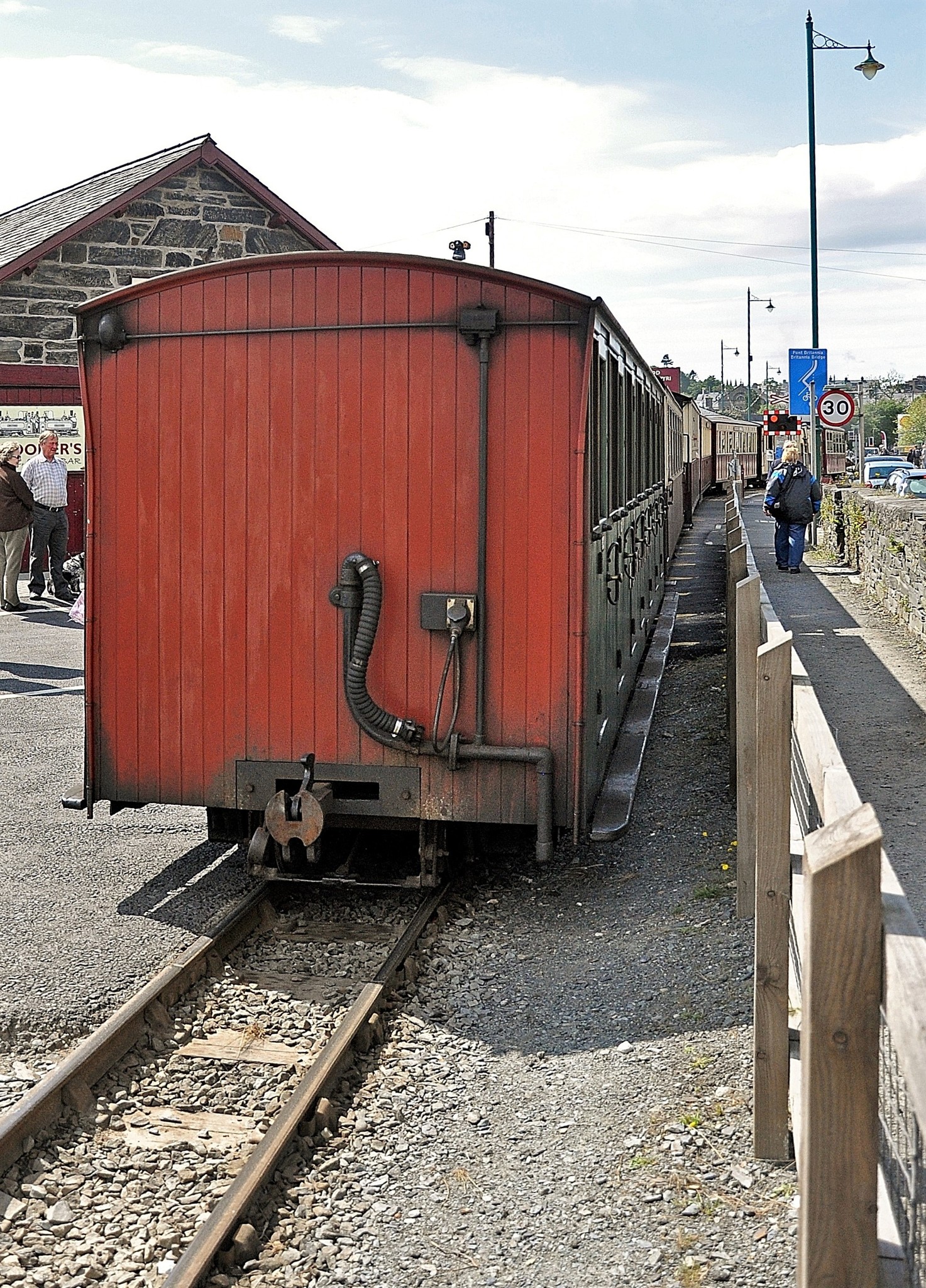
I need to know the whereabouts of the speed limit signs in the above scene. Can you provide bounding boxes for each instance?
[818,390,854,426]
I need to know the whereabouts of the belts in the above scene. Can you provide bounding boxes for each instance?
[33,501,64,512]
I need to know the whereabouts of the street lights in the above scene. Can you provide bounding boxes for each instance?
[721,339,739,411]
[748,286,777,423]
[802,7,887,490]
[764,360,781,414]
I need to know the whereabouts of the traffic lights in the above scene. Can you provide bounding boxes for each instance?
[761,411,801,436]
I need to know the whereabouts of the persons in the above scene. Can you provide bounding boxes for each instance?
[20,430,75,601]
[907,448,913,464]
[0,441,34,612]
[922,445,926,468]
[912,444,921,467]
[880,447,889,455]
[766,440,797,565]
[762,447,821,574]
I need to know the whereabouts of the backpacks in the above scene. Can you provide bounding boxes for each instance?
[915,449,920,457]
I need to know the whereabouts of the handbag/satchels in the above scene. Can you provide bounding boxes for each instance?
[768,495,786,516]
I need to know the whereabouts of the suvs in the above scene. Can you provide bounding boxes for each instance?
[882,468,926,499]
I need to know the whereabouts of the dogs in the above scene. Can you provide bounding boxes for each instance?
[46,550,86,599]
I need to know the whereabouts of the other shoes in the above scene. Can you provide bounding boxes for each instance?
[47,580,53,595]
[29,592,40,600]
[790,568,801,574]
[56,593,75,601]
[778,565,789,572]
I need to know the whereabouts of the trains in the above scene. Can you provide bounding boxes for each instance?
[61,208,847,886]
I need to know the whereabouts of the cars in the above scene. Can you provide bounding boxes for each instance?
[845,446,916,490]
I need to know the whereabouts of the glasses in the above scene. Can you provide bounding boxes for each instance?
[11,455,21,459]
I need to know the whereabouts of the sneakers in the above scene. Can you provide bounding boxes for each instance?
[4,602,28,611]
